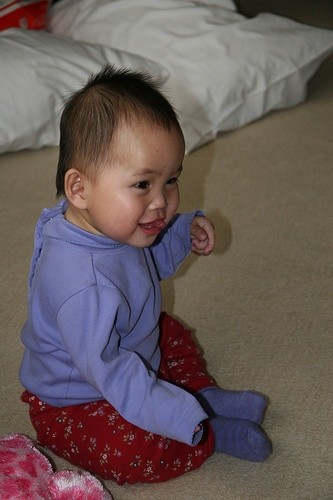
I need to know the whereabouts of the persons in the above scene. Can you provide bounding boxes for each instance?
[19,63,272,485]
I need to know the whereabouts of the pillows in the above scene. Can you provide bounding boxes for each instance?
[0,27,171,156]
[47,0,333,159]
